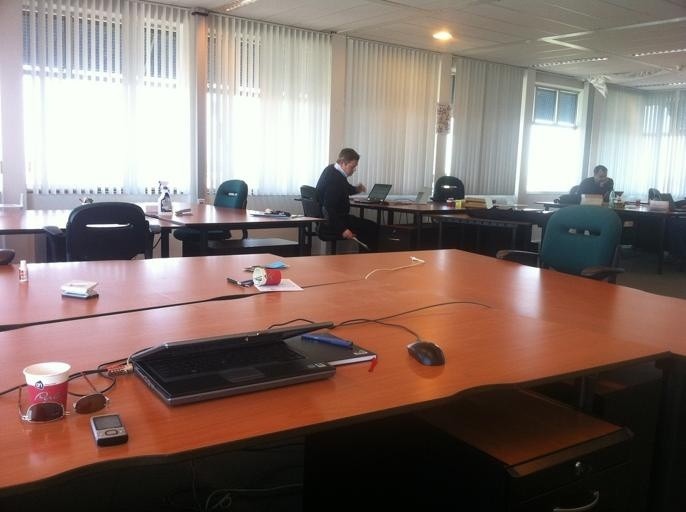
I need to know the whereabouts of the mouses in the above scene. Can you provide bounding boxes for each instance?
[378,201,389,206]
[408,340,445,366]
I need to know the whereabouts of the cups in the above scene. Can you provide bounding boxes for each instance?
[636,199,641,207]
[22,360,71,411]
[446,200,452,208]
[252,268,282,287]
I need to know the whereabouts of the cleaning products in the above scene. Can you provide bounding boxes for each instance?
[157,180,173,217]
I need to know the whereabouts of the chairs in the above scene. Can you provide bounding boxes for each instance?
[174,179,249,241]
[649,188,664,201]
[433,178,465,201]
[570,186,578,192]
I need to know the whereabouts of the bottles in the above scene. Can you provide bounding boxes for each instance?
[609,191,616,208]
[17,259,28,283]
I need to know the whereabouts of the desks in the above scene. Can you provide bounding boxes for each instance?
[0,202,686,511]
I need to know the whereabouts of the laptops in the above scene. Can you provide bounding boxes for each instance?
[360,184,392,204]
[604,191,624,202]
[131,321,335,407]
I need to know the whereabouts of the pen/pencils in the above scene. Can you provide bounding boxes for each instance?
[227,277,239,284]
[239,280,254,287]
[301,334,354,347]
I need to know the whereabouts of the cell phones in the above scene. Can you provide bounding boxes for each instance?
[90,412,128,447]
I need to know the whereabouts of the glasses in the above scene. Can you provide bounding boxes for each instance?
[17,371,107,424]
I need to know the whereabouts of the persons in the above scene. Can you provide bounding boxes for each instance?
[315,148,380,253]
[575,165,614,203]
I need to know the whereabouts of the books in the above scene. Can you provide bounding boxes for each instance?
[283,332,377,366]
